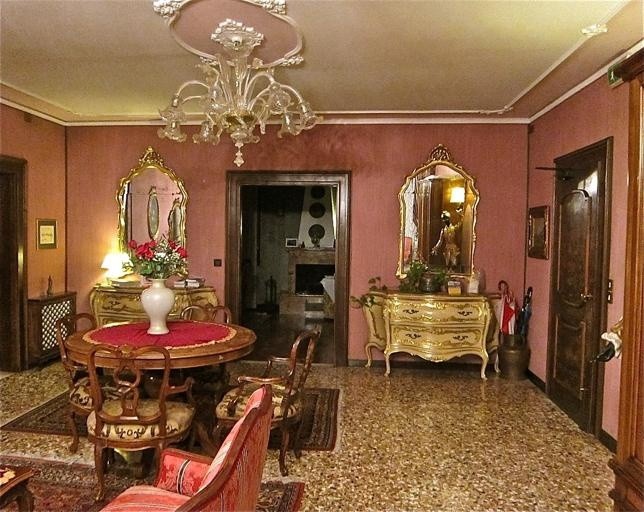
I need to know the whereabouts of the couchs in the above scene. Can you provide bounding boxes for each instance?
[97,382,274,512]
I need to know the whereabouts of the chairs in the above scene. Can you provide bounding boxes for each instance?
[54,312,133,454]
[179,304,235,320]
[213,322,322,475]
[85,343,194,500]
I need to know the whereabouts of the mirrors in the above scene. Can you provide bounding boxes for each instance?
[394,144,479,281]
[113,145,189,280]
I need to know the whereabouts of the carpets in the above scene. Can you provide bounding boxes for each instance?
[0,453,304,512]
[0,385,342,452]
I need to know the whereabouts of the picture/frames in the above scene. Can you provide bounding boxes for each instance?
[34,217,59,251]
[527,204,552,261]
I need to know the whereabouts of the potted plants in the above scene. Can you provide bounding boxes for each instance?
[399,261,450,294]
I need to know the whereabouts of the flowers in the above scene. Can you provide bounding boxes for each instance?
[127,239,188,279]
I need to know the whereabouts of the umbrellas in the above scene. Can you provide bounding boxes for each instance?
[517,287,534,333]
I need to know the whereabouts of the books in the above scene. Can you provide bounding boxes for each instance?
[174,277,204,288]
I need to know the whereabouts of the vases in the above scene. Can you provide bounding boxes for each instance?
[141,281,175,335]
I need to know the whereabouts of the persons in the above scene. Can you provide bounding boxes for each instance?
[493,280,516,334]
[432,208,464,267]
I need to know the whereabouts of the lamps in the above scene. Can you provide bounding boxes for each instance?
[153,0,323,169]
